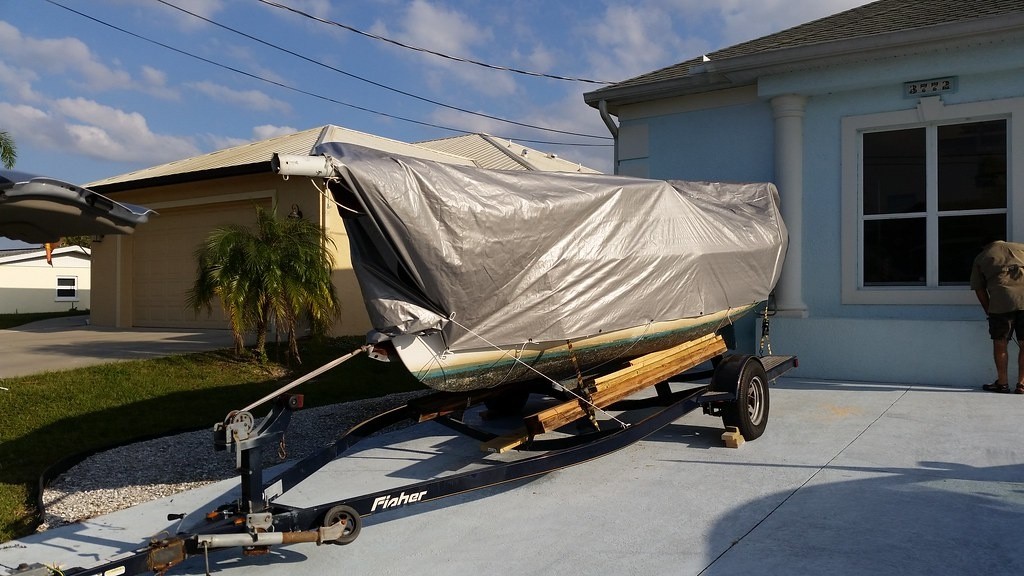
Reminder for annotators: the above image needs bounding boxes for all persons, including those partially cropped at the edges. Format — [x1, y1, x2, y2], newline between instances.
[970, 230, 1024, 394]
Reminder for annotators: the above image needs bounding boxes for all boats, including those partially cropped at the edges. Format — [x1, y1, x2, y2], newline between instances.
[267, 142, 790, 393]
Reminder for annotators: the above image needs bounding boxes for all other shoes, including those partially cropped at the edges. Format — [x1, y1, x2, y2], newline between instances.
[982, 380, 1010, 393]
[1014, 383, 1024, 393]
